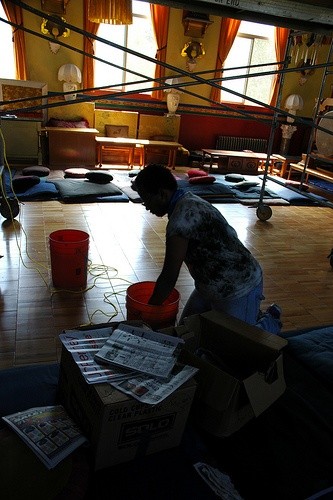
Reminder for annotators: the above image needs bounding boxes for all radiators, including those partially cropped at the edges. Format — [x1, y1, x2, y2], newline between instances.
[216, 136, 267, 152]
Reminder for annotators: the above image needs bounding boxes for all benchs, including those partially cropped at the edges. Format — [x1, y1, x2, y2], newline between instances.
[95, 136, 183, 170]
[270, 154, 301, 178]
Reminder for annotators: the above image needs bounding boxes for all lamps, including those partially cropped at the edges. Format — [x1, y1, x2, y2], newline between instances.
[284, 94, 304, 123]
[163, 78, 184, 114]
[40, 14, 70, 54]
[296, 58, 314, 87]
[181, 40, 206, 72]
[58, 64, 81, 100]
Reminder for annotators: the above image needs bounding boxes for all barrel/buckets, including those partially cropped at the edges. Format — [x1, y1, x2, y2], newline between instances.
[50, 230, 89, 291]
[127, 282, 180, 330]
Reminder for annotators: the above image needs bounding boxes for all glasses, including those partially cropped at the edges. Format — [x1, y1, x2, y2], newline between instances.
[142, 200, 153, 209]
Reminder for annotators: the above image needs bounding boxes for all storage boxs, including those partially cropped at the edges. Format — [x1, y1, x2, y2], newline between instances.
[157, 309, 288, 437]
[56, 320, 197, 471]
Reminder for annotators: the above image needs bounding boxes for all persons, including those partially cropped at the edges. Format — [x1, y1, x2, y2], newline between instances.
[130, 163, 283, 335]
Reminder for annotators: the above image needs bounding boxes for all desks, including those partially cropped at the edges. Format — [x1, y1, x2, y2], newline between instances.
[45, 127, 99, 169]
[202, 149, 278, 174]
[0, 116, 45, 166]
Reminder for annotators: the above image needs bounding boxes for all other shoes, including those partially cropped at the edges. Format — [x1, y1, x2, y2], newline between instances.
[266, 304, 283, 328]
